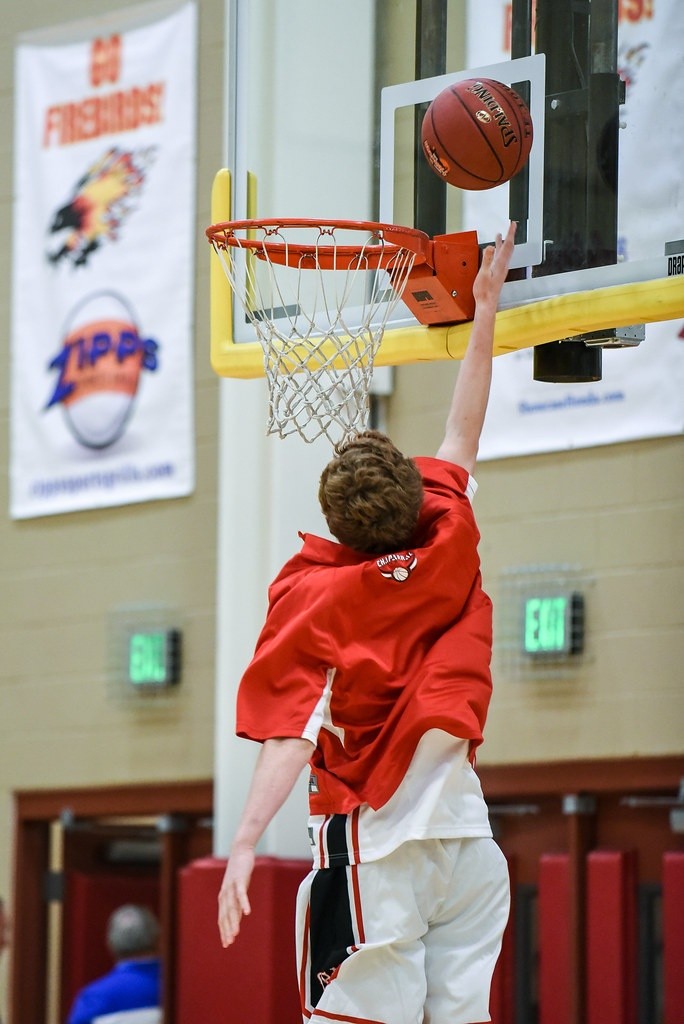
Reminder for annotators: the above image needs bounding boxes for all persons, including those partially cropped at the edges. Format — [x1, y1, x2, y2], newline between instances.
[218, 221, 518, 1024]
[68, 903, 162, 1024]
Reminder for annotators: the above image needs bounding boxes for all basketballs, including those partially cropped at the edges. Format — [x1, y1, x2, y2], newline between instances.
[421, 78, 534, 191]
